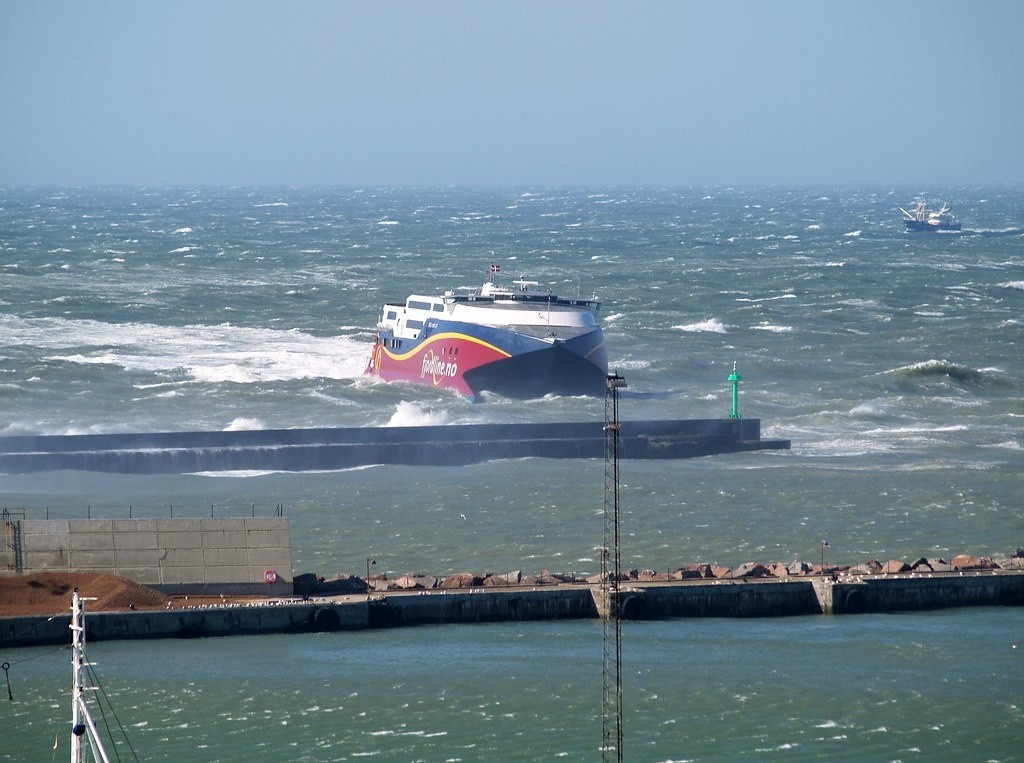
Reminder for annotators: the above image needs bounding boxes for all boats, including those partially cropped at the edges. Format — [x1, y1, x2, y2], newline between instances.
[899, 193, 962, 230]
[362, 261, 608, 405]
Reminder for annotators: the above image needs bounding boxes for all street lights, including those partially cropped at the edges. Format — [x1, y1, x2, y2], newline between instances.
[367, 557, 376, 600]
[821, 540, 829, 577]
